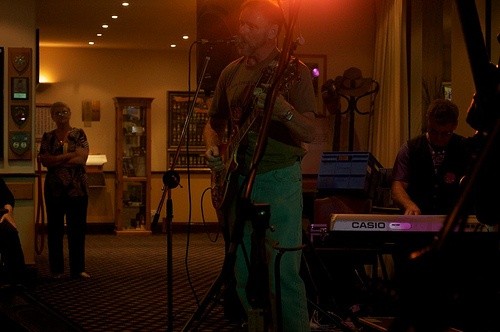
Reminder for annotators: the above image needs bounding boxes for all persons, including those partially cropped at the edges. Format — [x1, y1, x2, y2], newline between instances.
[201, 1, 320, 332]
[388, 99, 478, 320]
[0, 177, 28, 285]
[39, 101, 92, 284]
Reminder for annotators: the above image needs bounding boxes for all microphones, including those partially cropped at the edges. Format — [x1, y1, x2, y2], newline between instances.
[196, 36, 242, 46]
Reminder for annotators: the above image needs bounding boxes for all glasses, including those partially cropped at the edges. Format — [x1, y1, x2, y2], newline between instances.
[53, 111, 69, 116]
[427, 127, 453, 138]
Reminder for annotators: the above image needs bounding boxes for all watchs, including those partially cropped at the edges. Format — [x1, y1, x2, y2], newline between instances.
[278, 105, 296, 122]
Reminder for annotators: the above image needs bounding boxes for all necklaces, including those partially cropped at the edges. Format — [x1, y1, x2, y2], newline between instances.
[57, 135, 65, 144]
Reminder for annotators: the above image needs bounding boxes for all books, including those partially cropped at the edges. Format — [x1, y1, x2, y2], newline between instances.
[171, 101, 210, 168]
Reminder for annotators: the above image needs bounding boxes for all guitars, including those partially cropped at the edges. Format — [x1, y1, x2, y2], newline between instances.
[210, 55, 302, 211]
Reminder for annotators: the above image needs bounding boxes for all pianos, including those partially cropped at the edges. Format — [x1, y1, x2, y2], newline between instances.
[328, 212, 500, 233]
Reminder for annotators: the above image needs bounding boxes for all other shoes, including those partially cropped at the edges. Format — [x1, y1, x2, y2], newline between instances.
[70, 272, 90, 278]
[47, 274, 60, 279]
[4, 273, 24, 288]
